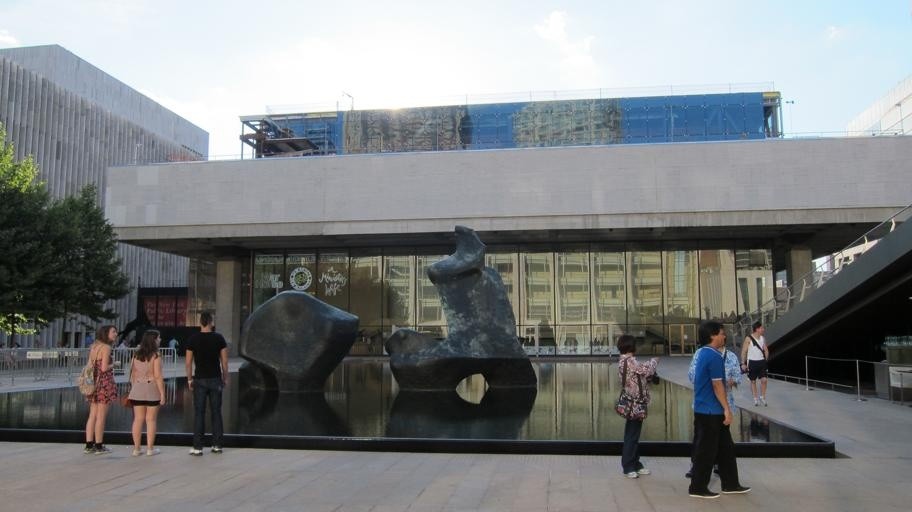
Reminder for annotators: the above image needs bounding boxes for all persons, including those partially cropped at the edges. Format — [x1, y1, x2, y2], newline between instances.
[124, 328, 166, 456]
[614, 334, 662, 478]
[686, 319, 752, 499]
[167, 337, 179, 362]
[185, 310, 228, 456]
[739, 320, 770, 407]
[79, 325, 123, 456]
[681, 318, 742, 478]
[0, 332, 140, 370]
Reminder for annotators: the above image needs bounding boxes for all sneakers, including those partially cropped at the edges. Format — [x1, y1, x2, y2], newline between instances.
[685, 469, 692, 478]
[712, 468, 720, 477]
[754, 399, 758, 406]
[211, 446, 222, 453]
[188, 447, 203, 456]
[623, 472, 639, 480]
[636, 468, 651, 475]
[688, 485, 720, 499]
[133, 449, 144, 457]
[146, 448, 161, 456]
[720, 486, 752, 495]
[760, 396, 767, 407]
[82, 446, 96, 454]
[94, 447, 112, 455]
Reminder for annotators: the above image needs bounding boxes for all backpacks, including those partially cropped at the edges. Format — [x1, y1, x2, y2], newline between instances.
[79, 341, 104, 397]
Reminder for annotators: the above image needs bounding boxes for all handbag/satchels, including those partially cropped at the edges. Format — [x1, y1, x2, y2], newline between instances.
[120, 392, 133, 408]
[615, 389, 647, 420]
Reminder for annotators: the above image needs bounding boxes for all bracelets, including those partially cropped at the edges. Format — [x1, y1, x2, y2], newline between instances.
[188, 378, 194, 383]
[740, 362, 746, 366]
[113, 362, 117, 368]
